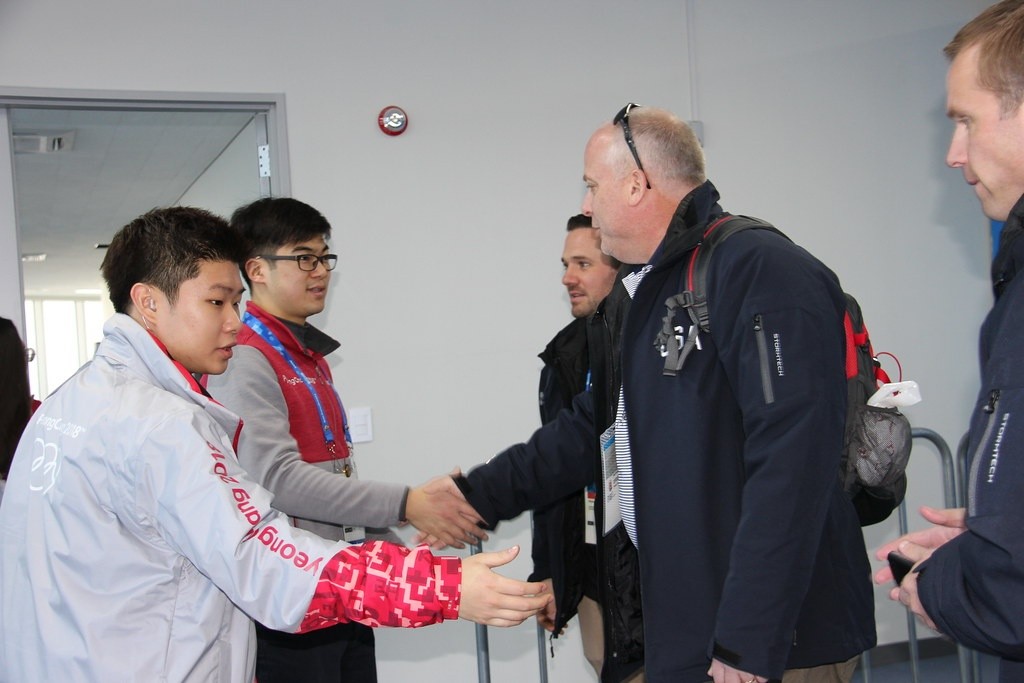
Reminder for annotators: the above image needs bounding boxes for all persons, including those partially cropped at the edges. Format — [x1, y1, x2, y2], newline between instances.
[200, 199, 489, 683]
[0, 208, 554, 683]
[873, 0, 1024, 683]
[525, 214, 647, 683]
[413, 102, 878, 683]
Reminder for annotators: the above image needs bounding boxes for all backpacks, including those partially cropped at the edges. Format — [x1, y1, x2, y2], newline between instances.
[684, 214, 912, 528]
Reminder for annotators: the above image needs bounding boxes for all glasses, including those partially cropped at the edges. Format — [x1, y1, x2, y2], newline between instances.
[614, 102, 651, 190]
[246, 253, 338, 272]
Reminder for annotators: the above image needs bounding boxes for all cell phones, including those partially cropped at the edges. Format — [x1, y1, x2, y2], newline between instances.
[888, 550, 915, 587]
